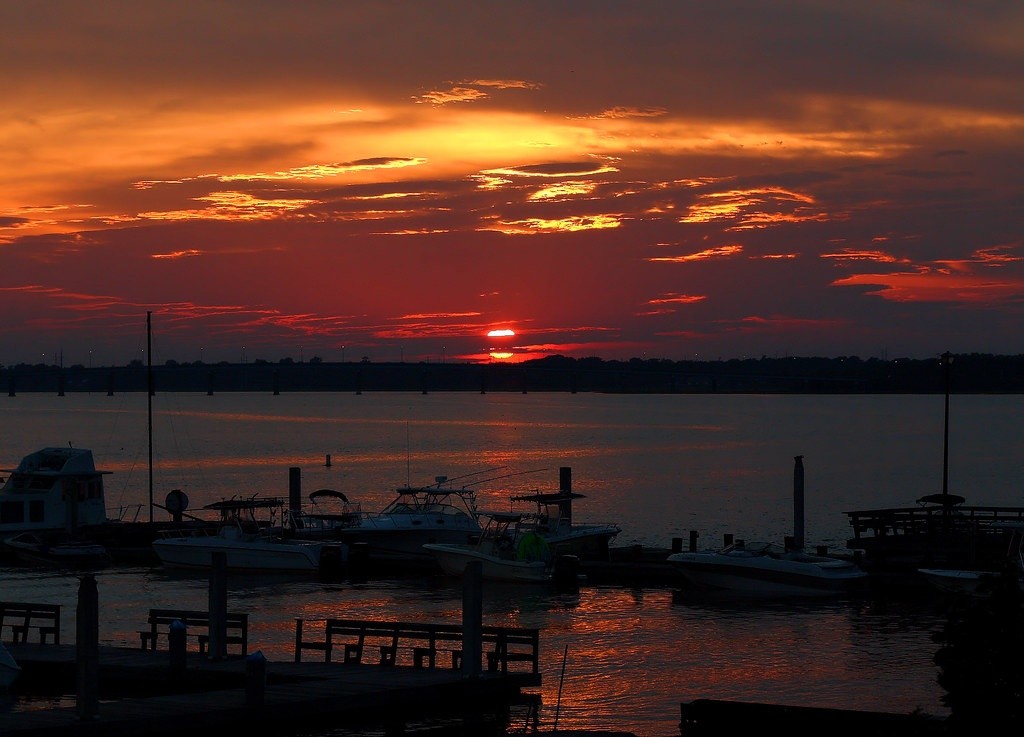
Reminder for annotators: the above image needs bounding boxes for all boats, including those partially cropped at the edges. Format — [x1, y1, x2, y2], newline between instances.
[152, 453, 856, 608]
